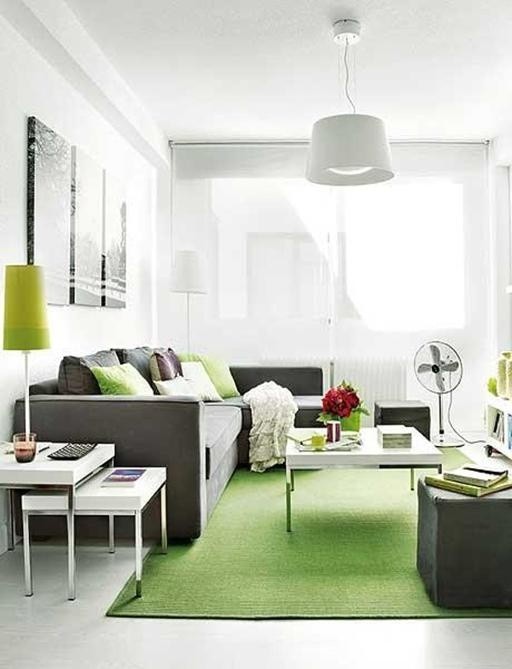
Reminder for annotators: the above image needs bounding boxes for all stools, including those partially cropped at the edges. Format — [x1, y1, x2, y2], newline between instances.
[417, 473, 512, 610]
[374, 400, 430, 467]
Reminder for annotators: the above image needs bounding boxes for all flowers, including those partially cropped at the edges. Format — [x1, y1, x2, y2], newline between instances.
[317, 380, 371, 427]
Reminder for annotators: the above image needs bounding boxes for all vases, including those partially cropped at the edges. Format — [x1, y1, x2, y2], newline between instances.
[341, 413, 361, 431]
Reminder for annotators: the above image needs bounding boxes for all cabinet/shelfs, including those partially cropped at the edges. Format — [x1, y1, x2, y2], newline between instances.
[485, 398, 512, 460]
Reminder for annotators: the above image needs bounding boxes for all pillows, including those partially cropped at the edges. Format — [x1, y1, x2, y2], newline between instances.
[150, 348, 183, 380]
[90, 362, 155, 396]
[112, 346, 155, 387]
[180, 361, 224, 403]
[58, 350, 120, 396]
[179, 352, 241, 397]
[152, 375, 202, 400]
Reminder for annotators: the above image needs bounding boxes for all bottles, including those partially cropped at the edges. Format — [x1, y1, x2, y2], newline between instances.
[495, 349, 512, 400]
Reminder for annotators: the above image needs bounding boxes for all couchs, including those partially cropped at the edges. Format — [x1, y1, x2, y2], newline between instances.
[14, 365, 324, 545]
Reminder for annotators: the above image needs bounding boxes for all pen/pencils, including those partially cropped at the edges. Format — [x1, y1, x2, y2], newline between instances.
[39, 447, 51, 452]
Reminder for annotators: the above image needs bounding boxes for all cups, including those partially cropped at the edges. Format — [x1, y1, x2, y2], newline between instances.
[13, 432, 36, 463]
[311, 434, 325, 450]
[326, 420, 342, 443]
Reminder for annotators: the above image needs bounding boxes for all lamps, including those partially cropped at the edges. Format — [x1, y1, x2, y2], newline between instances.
[171, 248, 208, 354]
[305, 18, 394, 186]
[3, 264, 52, 443]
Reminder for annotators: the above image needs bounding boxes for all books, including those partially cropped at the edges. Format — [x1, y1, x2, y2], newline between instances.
[97, 464, 150, 486]
[285, 428, 363, 452]
[492, 410, 512, 450]
[425, 472, 512, 496]
[376, 423, 411, 449]
[443, 462, 510, 488]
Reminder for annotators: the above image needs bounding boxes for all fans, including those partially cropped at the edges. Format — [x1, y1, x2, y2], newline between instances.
[413, 341, 465, 448]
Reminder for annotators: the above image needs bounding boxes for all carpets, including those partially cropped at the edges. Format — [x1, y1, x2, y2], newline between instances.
[104, 444, 512, 617]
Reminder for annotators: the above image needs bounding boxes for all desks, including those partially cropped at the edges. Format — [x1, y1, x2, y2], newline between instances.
[20, 466, 169, 600]
[0, 440, 113, 603]
